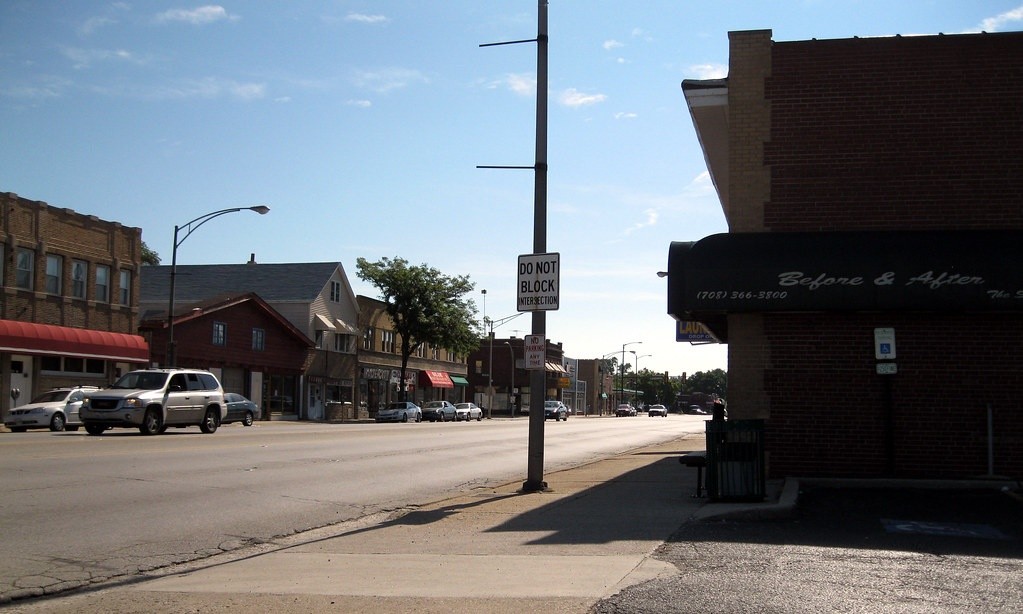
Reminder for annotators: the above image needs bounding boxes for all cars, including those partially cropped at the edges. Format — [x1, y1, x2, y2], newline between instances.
[544, 400, 568, 421]
[223, 392, 259, 427]
[615, 404, 637, 416]
[375, 402, 421, 423]
[648, 404, 668, 417]
[453, 402, 483, 421]
[422, 401, 456, 422]
[5, 386, 105, 433]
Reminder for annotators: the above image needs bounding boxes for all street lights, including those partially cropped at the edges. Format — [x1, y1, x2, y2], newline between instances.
[621, 340, 642, 405]
[599, 351, 636, 415]
[165, 205, 270, 366]
[634, 354, 652, 404]
[503, 341, 514, 418]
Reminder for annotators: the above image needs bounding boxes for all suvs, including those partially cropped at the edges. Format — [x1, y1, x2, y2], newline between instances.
[79, 367, 227, 435]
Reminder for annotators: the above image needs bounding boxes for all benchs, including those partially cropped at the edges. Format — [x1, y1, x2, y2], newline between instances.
[679, 450, 706, 498]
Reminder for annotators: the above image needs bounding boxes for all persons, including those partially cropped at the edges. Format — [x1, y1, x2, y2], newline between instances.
[419, 400, 424, 409]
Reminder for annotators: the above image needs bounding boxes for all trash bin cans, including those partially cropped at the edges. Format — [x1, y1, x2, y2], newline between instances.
[706, 420, 769, 503]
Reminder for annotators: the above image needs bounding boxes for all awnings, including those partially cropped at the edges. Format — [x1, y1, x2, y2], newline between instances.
[0, 319, 149, 362]
[335, 319, 361, 334]
[545, 363, 566, 373]
[419, 370, 453, 388]
[315, 315, 336, 330]
[450, 377, 469, 387]
